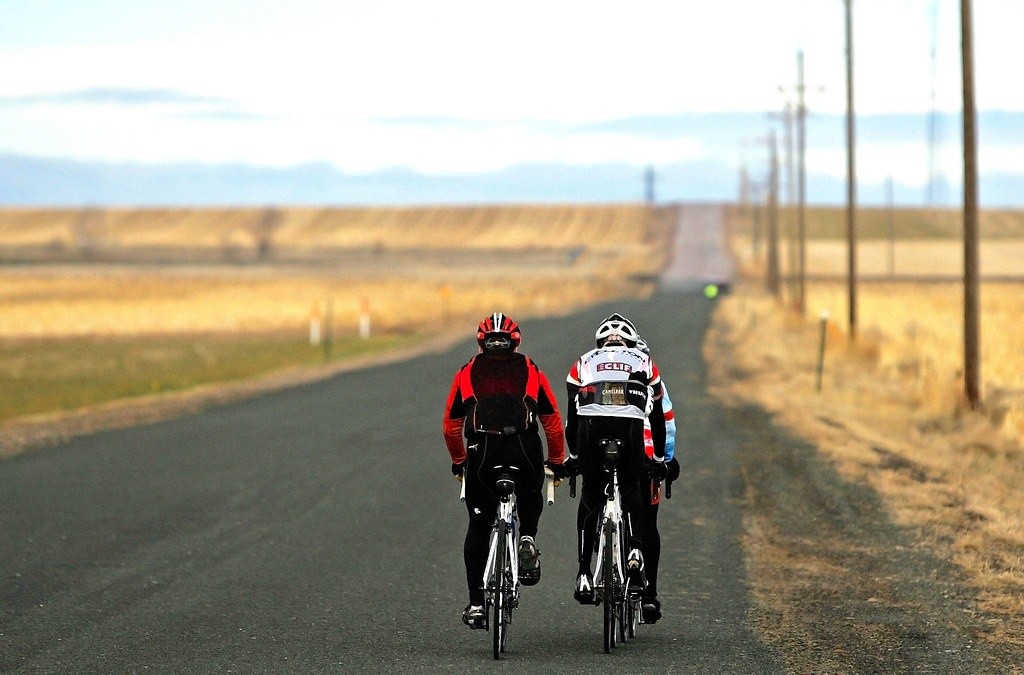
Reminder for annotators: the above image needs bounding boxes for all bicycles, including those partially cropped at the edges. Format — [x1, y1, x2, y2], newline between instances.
[565, 430, 684, 655]
[450, 453, 557, 662]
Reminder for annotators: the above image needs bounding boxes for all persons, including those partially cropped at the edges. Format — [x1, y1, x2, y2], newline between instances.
[442, 312, 564, 627]
[565, 313, 680, 623]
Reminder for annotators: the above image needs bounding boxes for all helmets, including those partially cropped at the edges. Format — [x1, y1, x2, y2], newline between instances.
[595, 313, 637, 348]
[637, 335, 650, 353]
[477, 312, 521, 352]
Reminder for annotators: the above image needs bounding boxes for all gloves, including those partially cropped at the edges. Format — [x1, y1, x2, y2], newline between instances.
[544, 460, 565, 487]
[562, 456, 582, 477]
[451, 461, 466, 482]
[646, 458, 667, 482]
[666, 456, 680, 484]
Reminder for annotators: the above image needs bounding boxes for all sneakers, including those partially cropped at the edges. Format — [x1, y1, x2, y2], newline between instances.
[574, 573, 594, 603]
[642, 601, 662, 623]
[462, 603, 486, 628]
[519, 534, 541, 586]
[627, 548, 647, 600]
